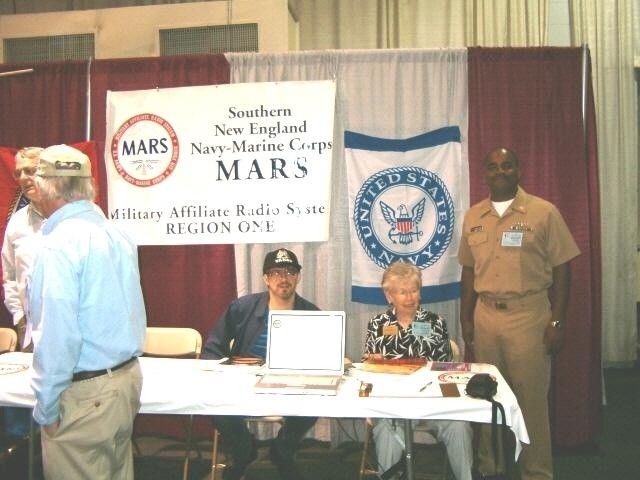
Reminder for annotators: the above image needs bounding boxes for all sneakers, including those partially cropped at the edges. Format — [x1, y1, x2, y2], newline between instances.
[222, 449, 261, 480]
[270, 441, 303, 480]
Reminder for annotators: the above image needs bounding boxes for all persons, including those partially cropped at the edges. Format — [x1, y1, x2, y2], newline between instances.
[23, 143, 149, 480]
[459, 146, 583, 479]
[1, 146, 48, 353]
[202, 248, 333, 480]
[363, 260, 478, 480]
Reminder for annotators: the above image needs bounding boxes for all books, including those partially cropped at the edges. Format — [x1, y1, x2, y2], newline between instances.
[430, 359, 473, 374]
[363, 354, 427, 375]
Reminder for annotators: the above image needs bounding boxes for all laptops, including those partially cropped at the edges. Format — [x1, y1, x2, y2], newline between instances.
[254, 310, 347, 396]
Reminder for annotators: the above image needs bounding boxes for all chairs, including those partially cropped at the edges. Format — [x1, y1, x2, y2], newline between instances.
[1, 327, 41, 479]
[132, 326, 203, 479]
[359, 341, 461, 479]
[211, 339, 285, 477]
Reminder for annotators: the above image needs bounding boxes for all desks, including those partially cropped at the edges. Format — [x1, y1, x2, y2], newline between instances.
[0, 353, 498, 476]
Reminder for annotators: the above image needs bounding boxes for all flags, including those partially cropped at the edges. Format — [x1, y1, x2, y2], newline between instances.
[2, 140, 100, 299]
[102, 80, 335, 244]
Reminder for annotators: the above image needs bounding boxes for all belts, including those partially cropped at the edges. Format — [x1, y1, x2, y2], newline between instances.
[74, 356, 137, 381]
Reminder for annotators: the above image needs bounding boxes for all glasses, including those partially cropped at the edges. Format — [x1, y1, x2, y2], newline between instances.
[265, 270, 298, 276]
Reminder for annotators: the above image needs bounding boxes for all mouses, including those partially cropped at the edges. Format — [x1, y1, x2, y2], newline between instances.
[360, 383, 373, 397]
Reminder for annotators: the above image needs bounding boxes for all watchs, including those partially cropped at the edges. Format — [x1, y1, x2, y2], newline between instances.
[550, 321, 563, 330]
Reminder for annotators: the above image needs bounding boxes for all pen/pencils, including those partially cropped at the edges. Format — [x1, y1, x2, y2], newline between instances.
[420, 382, 432, 392]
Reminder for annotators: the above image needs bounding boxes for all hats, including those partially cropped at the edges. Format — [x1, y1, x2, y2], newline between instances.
[36, 144, 91, 177]
[264, 249, 301, 273]
